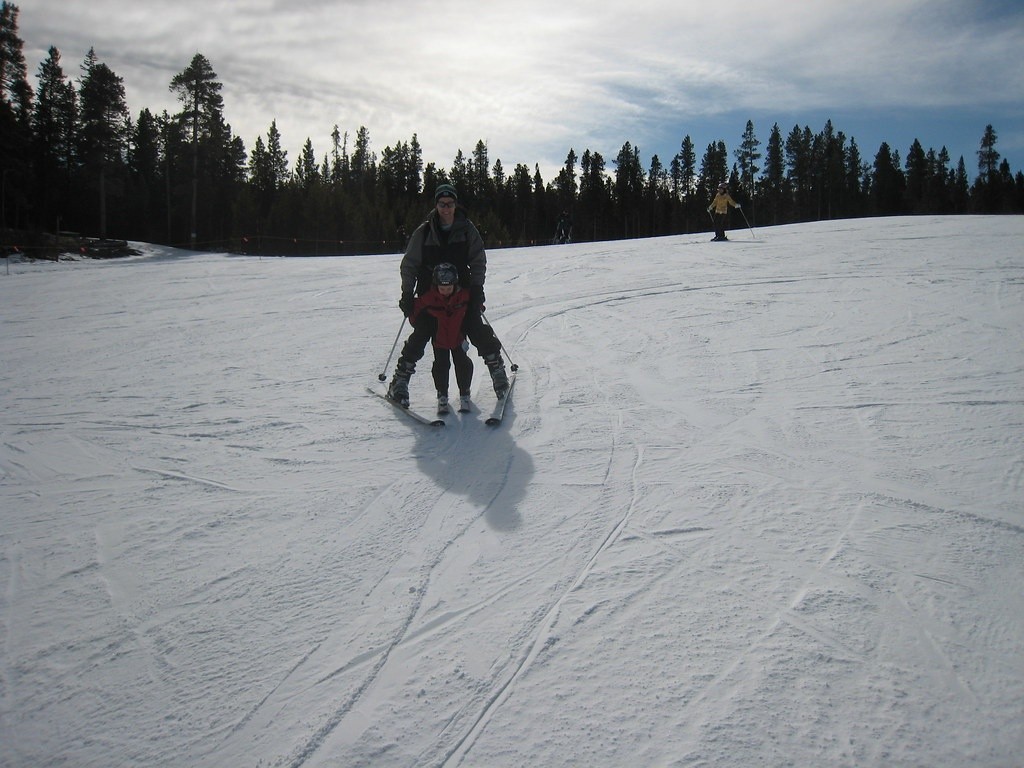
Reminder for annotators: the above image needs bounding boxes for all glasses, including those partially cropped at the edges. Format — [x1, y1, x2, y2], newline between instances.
[437, 200, 456, 208]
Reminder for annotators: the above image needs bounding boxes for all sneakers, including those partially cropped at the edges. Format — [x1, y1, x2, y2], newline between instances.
[437, 395, 449, 414]
[458, 394, 471, 413]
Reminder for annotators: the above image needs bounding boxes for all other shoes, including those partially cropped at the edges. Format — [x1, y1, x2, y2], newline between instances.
[715, 235, 725, 239]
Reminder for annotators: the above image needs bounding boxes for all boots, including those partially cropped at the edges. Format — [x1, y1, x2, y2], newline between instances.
[389, 356, 416, 408]
[485, 352, 510, 392]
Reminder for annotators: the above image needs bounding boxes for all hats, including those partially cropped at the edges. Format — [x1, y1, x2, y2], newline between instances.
[435, 184, 458, 206]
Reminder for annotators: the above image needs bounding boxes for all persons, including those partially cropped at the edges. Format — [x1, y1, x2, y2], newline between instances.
[407, 261, 487, 415]
[706, 183, 741, 241]
[389, 185, 511, 409]
[554, 209, 574, 244]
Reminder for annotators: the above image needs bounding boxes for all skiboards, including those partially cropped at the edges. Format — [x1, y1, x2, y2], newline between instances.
[366, 379, 447, 428]
[485, 375, 516, 426]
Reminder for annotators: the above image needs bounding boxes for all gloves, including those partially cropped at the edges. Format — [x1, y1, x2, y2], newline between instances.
[399, 292, 414, 317]
[735, 204, 741, 208]
[707, 206, 712, 210]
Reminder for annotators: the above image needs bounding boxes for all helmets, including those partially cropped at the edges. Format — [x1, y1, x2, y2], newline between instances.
[432, 262, 458, 287]
[718, 183, 727, 188]
